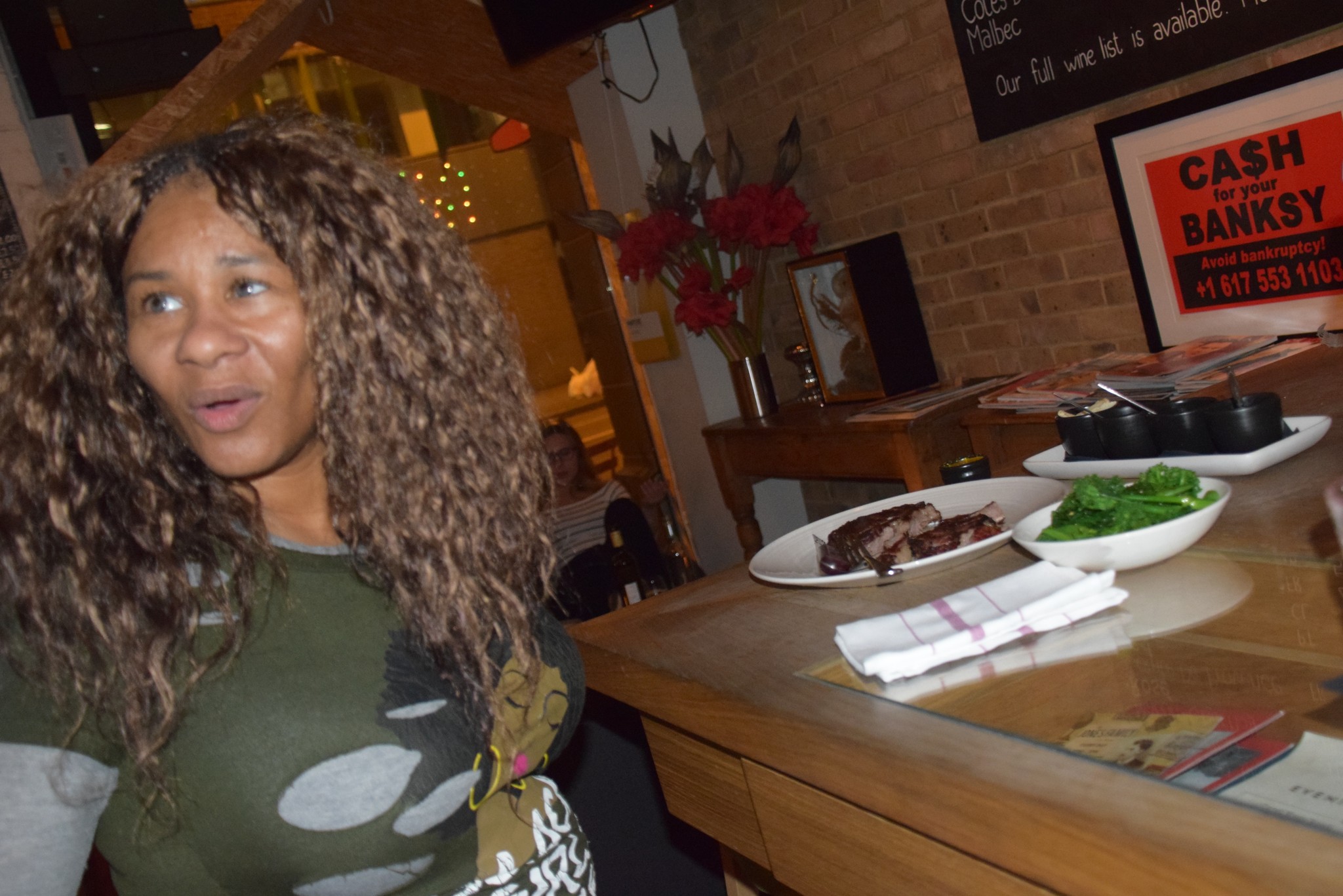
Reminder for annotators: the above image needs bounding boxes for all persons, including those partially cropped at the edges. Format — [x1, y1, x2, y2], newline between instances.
[539, 421, 666, 575]
[1, 105, 599, 896]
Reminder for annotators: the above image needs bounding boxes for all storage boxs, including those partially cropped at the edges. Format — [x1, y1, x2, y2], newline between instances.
[787, 233, 939, 404]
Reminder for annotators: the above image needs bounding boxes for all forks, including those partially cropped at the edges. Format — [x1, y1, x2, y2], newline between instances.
[842, 539, 904, 578]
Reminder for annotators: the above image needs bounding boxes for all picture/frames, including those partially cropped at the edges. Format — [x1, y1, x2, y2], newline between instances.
[1093, 44, 1343, 354]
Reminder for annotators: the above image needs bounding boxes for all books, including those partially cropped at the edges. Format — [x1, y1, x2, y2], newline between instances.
[976, 333, 1323, 414]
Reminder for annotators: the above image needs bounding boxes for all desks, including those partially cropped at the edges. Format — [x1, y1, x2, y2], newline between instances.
[963, 338, 1327, 478]
[703, 375, 1026, 558]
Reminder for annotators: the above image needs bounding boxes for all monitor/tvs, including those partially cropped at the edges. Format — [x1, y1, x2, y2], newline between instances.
[480, 0, 680, 68]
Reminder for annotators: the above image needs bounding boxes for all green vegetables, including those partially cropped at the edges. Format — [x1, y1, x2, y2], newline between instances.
[1036, 462, 1222, 540]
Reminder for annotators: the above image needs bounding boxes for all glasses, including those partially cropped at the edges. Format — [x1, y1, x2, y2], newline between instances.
[547, 448, 575, 463]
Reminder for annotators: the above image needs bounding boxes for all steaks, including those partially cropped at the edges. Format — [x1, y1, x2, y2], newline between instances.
[823, 501, 1006, 568]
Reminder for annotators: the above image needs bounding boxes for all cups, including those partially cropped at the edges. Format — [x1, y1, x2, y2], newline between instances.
[1146, 396, 1215, 456]
[1214, 391, 1283, 455]
[1094, 400, 1146, 459]
[1055, 403, 1114, 461]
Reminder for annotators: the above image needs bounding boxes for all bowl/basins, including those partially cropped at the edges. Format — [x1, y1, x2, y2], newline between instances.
[1011, 477, 1233, 571]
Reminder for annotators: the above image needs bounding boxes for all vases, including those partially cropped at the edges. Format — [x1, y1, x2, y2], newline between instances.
[728, 354, 777, 419]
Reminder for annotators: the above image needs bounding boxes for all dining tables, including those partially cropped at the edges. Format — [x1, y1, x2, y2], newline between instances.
[570, 337, 1343, 896]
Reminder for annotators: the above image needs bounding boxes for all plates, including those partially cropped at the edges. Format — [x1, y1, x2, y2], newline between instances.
[1021, 415, 1331, 476]
[748, 476, 1067, 587]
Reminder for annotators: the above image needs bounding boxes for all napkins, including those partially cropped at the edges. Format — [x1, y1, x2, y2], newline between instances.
[833, 560, 1127, 685]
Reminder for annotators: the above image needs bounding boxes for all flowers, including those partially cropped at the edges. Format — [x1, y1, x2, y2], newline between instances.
[571, 113, 819, 360]
